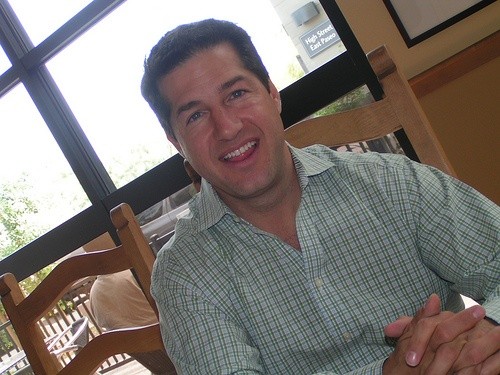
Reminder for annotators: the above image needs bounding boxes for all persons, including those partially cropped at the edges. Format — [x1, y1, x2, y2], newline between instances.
[141, 18, 500, 375]
[82, 231, 159, 331]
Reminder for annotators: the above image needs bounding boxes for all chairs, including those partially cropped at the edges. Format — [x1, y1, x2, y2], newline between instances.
[0, 42, 459, 375]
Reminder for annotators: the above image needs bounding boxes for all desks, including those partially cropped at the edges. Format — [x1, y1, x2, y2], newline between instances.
[0, 334, 57, 375]
